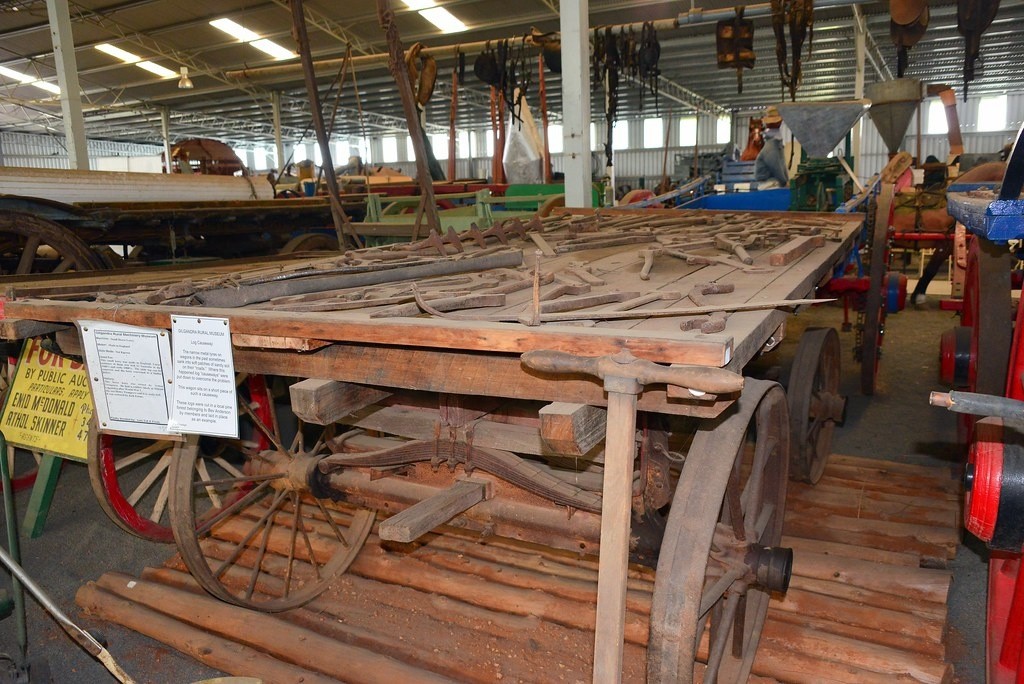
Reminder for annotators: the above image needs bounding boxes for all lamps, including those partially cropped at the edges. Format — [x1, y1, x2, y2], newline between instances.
[177, 67, 194, 88]
